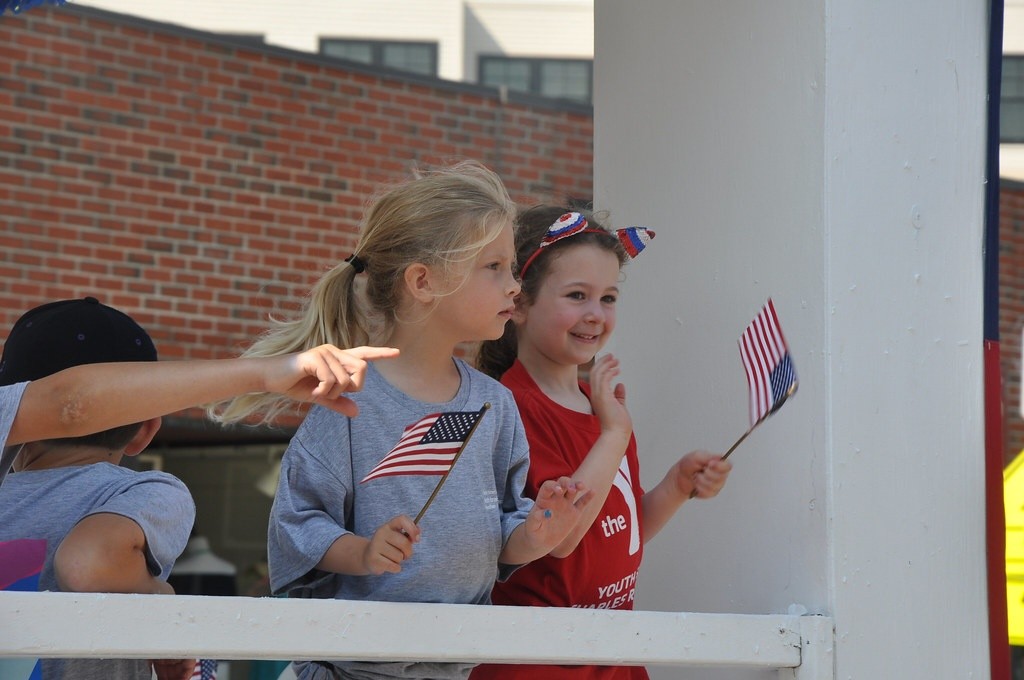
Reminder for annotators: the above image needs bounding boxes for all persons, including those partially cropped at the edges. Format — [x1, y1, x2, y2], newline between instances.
[468, 193, 735, 680]
[0, 288, 200, 680]
[189, 155, 596, 679]
[0, 334, 408, 458]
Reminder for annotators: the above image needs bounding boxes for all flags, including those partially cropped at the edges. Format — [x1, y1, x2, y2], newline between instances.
[735, 296, 798, 427]
